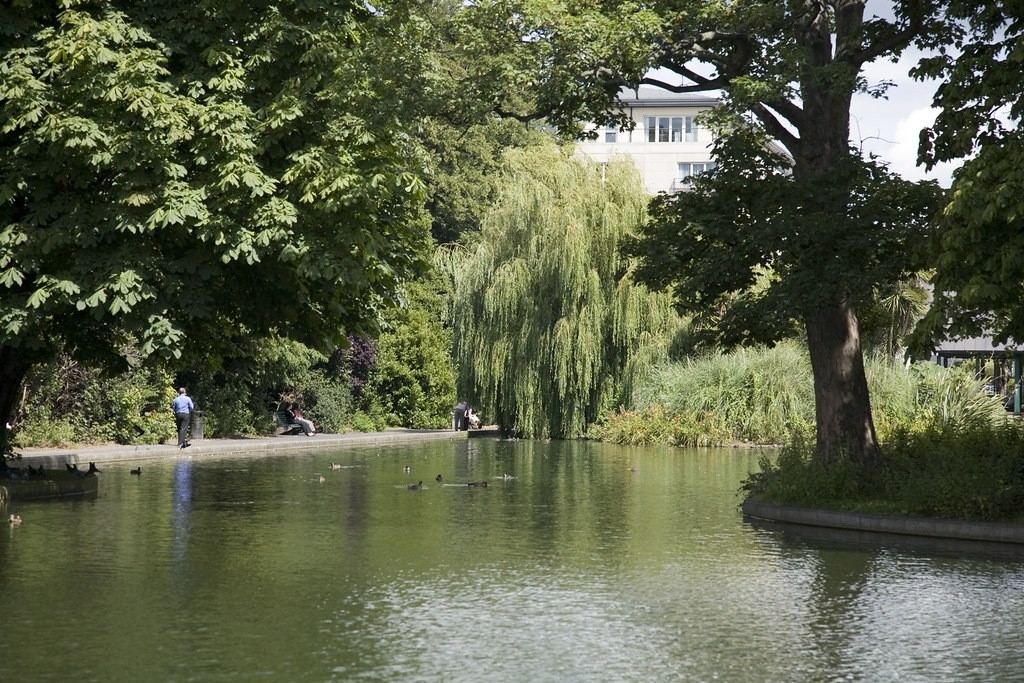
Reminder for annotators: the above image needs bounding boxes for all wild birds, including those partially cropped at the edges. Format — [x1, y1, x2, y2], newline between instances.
[468, 481, 489, 488]
[7, 514, 22, 524]
[318, 475, 324, 481]
[4, 462, 143, 481]
[435, 474, 442, 481]
[329, 462, 340, 470]
[406, 481, 423, 489]
[403, 465, 411, 472]
[631, 465, 637, 471]
[502, 473, 513, 480]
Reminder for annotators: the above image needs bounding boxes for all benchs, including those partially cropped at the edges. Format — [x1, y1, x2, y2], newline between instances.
[274, 411, 301, 435]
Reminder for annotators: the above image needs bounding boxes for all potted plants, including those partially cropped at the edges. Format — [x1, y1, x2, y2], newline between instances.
[189, 393, 204, 439]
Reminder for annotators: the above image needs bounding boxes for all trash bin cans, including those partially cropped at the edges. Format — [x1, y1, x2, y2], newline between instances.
[190, 411, 205, 437]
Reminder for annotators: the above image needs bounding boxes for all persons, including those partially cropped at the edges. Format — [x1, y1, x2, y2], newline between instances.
[172, 388, 194, 449]
[454, 401, 480, 431]
[286, 402, 316, 437]
